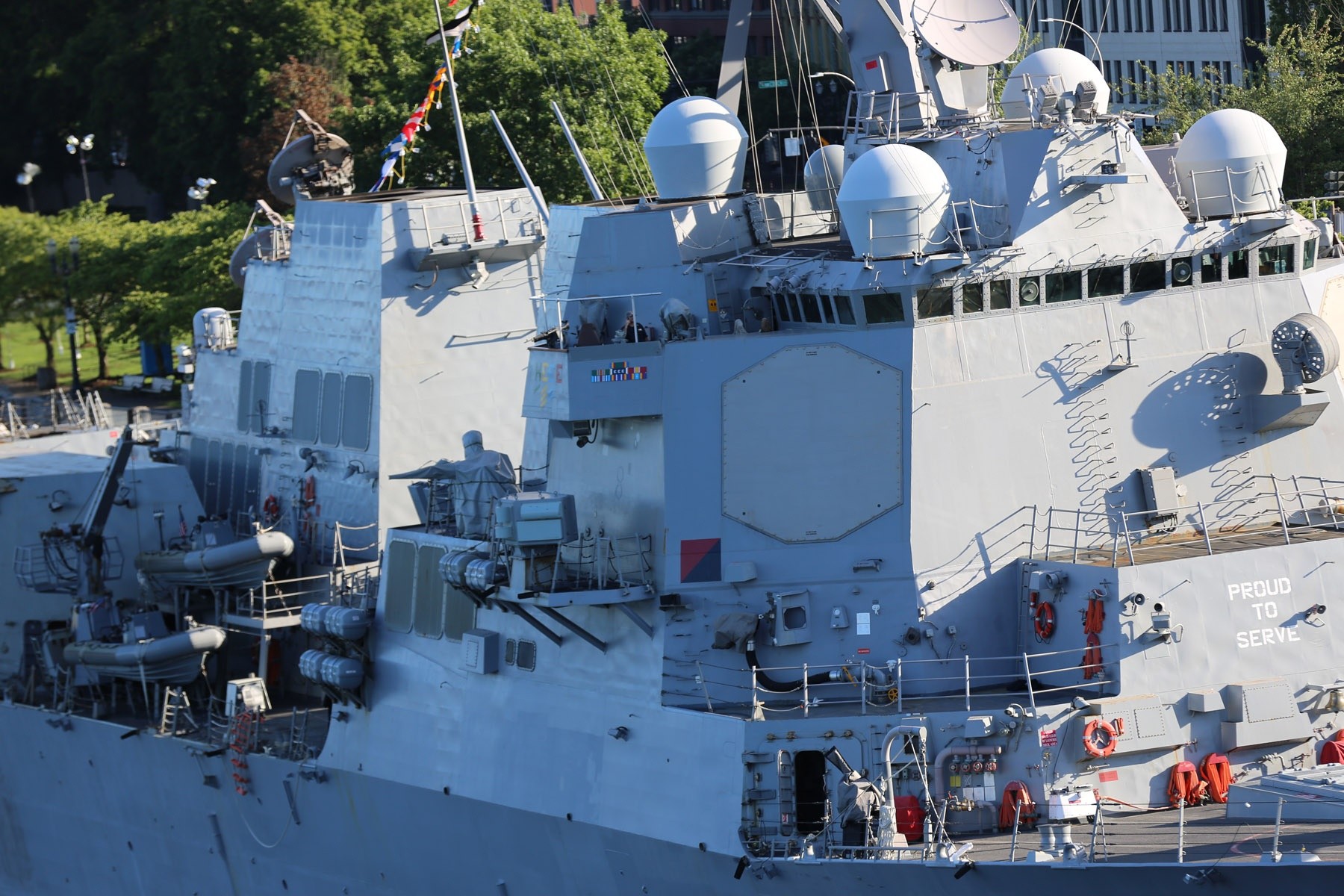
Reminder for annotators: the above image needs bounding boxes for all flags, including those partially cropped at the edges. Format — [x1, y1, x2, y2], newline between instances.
[369, 0, 482, 192]
[179, 509, 188, 537]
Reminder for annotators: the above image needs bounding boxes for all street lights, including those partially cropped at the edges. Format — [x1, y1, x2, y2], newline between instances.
[65, 133, 95, 203]
[188, 178, 215, 207]
[17, 162, 39, 213]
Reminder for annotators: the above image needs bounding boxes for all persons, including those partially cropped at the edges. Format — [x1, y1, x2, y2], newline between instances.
[5, 673, 29, 703]
[620, 311, 648, 343]
[758, 318, 772, 333]
[1214, 253, 1221, 279]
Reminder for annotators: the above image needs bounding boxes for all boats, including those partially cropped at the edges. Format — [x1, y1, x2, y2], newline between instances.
[133, 508, 296, 593]
[0, 0, 1343, 895]
[59, 601, 225, 686]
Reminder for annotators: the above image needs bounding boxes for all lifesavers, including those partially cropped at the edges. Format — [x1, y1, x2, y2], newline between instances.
[1084, 719, 1117, 757]
[1036, 601, 1053, 637]
[264, 495, 279, 524]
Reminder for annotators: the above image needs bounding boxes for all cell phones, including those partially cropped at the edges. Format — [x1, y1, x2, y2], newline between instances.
[626, 317, 629, 320]
